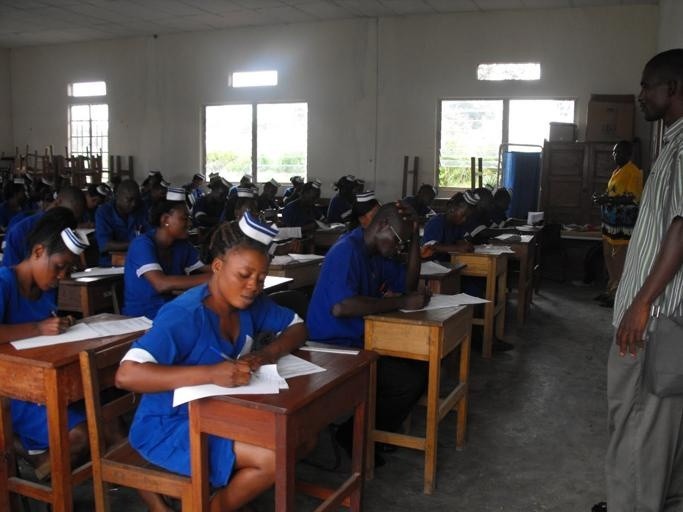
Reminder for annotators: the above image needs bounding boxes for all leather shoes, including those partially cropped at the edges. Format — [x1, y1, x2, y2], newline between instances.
[335, 423, 385, 471]
[377, 443, 399, 453]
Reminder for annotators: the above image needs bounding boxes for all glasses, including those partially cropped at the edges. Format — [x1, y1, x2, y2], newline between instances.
[388, 226, 413, 250]
[165, 214, 194, 225]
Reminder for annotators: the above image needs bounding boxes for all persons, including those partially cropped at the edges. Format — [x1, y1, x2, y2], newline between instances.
[306, 199, 433, 467]
[257, 180, 281, 210]
[1, 207, 177, 511]
[326, 175, 353, 222]
[406, 184, 437, 219]
[85, 183, 111, 209]
[592, 49, 683, 512]
[283, 179, 329, 233]
[115, 208, 308, 512]
[0, 186, 88, 267]
[122, 188, 212, 320]
[423, 189, 480, 253]
[181, 174, 205, 209]
[143, 170, 163, 191]
[0, 177, 25, 233]
[284, 176, 304, 201]
[592, 139, 643, 307]
[486, 188, 511, 230]
[95, 179, 153, 268]
[193, 178, 233, 230]
[337, 191, 382, 242]
[142, 181, 168, 222]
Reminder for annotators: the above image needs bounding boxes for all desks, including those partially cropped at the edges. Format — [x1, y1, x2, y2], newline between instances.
[401, 156, 482, 212]
[314, 228, 346, 246]
[109, 251, 128, 265]
[560, 227, 605, 265]
[473, 235, 536, 336]
[0, 313, 147, 512]
[417, 262, 467, 294]
[363, 292, 474, 495]
[267, 253, 326, 292]
[0, 146, 134, 189]
[188, 340, 379, 512]
[505, 223, 545, 294]
[171, 271, 294, 307]
[273, 238, 302, 255]
[58, 275, 111, 318]
[450, 251, 508, 359]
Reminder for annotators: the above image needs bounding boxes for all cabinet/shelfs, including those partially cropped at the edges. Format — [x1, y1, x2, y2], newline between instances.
[536, 139, 640, 235]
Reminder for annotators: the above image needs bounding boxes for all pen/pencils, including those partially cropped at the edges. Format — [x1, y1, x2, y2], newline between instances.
[49, 310, 58, 316]
[210, 346, 258, 379]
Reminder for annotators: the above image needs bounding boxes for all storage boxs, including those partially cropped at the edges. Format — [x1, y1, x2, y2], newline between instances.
[549, 122, 577, 144]
[584, 92, 635, 142]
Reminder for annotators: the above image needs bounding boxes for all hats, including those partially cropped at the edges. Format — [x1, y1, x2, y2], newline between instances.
[356, 189, 377, 203]
[165, 184, 187, 202]
[239, 209, 282, 248]
[195, 171, 206, 181]
[148, 169, 157, 178]
[355, 179, 366, 185]
[345, 174, 356, 183]
[96, 184, 110, 199]
[270, 179, 281, 189]
[61, 226, 91, 256]
[235, 187, 255, 198]
[218, 179, 233, 188]
[312, 177, 322, 190]
[462, 187, 480, 206]
[160, 180, 171, 188]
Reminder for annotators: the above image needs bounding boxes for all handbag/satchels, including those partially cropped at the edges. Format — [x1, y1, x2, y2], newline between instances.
[638, 143, 683, 399]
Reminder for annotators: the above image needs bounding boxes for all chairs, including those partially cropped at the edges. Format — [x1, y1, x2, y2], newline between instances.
[79, 331, 192, 512]
[267, 290, 307, 324]
[111, 274, 125, 315]
